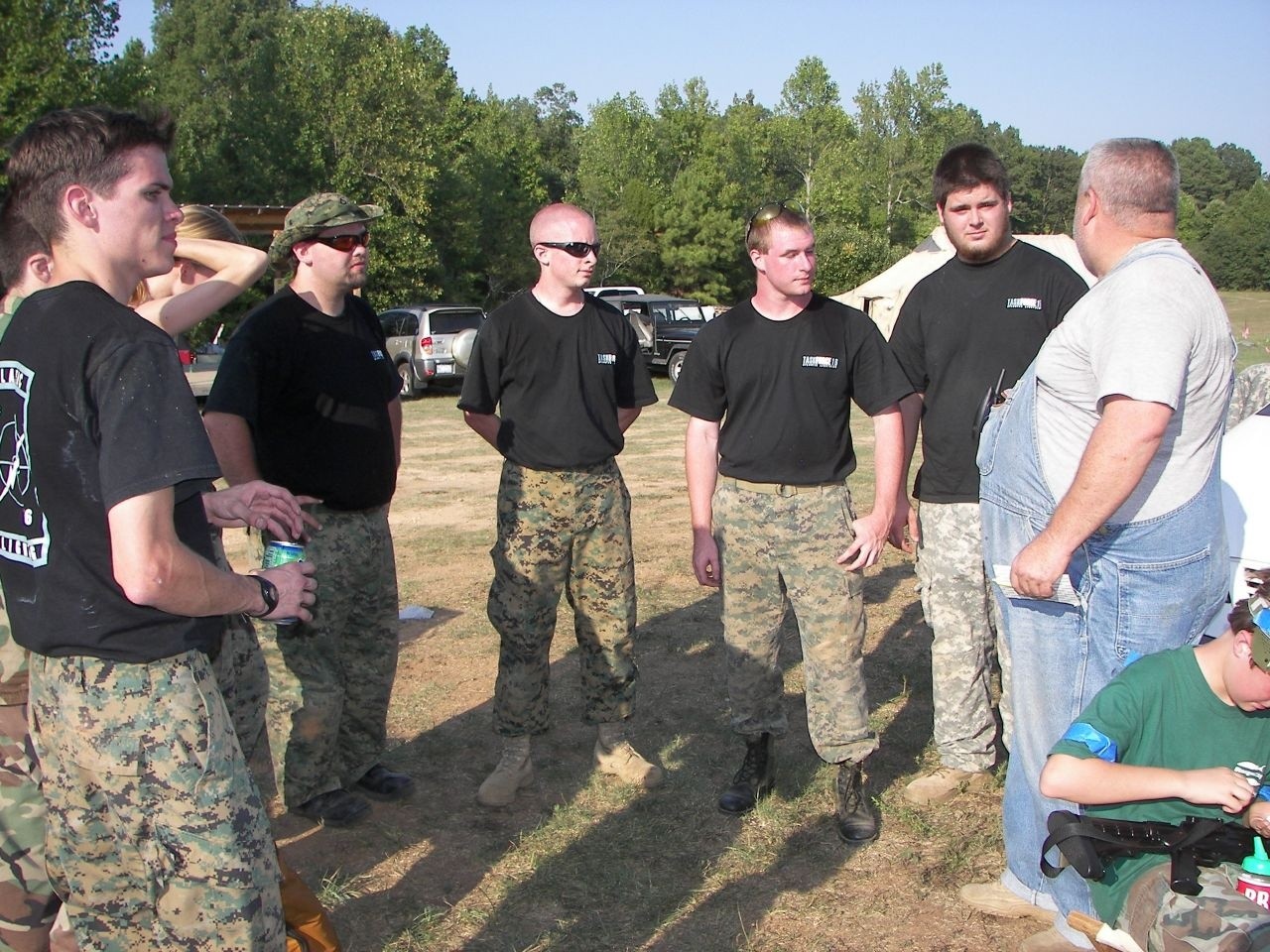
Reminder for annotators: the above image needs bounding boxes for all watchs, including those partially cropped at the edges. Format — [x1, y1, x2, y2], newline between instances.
[245, 576, 278, 619]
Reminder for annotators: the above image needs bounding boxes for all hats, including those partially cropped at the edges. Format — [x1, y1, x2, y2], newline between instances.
[268, 192, 385, 271]
[1246, 593, 1270, 671]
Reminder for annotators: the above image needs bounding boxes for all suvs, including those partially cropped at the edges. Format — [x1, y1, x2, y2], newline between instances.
[598, 292, 723, 385]
[377, 303, 489, 401]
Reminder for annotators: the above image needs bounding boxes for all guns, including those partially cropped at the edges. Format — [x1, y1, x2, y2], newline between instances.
[1045, 808, 1270, 897]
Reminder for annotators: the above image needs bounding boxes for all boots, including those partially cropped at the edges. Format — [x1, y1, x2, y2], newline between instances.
[714, 733, 770, 814]
[592, 721, 664, 786]
[479, 732, 533, 807]
[835, 763, 876, 843]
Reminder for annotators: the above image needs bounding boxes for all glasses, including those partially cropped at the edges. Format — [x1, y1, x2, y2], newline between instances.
[535, 242, 601, 260]
[744, 200, 804, 246]
[305, 230, 371, 250]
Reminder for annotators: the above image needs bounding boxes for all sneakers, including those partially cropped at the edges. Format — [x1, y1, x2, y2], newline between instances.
[961, 881, 1059, 924]
[903, 765, 991, 804]
[354, 767, 415, 798]
[1019, 926, 1094, 952]
[290, 789, 372, 827]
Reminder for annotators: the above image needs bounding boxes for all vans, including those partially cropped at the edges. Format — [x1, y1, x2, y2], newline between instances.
[582, 286, 646, 317]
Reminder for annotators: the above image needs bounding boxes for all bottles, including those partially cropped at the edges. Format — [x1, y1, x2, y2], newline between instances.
[1236, 836, 1270, 913]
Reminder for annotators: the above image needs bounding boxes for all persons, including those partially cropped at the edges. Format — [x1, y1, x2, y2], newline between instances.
[959, 137, 1238, 952]
[668, 197, 915, 845]
[0, 101, 319, 952]
[1040, 580, 1270, 952]
[201, 194, 416, 823]
[456, 203, 667, 807]
[887, 145, 1090, 804]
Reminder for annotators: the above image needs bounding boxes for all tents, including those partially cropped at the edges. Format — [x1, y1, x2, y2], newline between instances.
[827, 226, 1099, 343]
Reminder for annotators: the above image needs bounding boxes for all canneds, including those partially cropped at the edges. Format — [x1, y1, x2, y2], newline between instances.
[262, 540, 304, 625]
